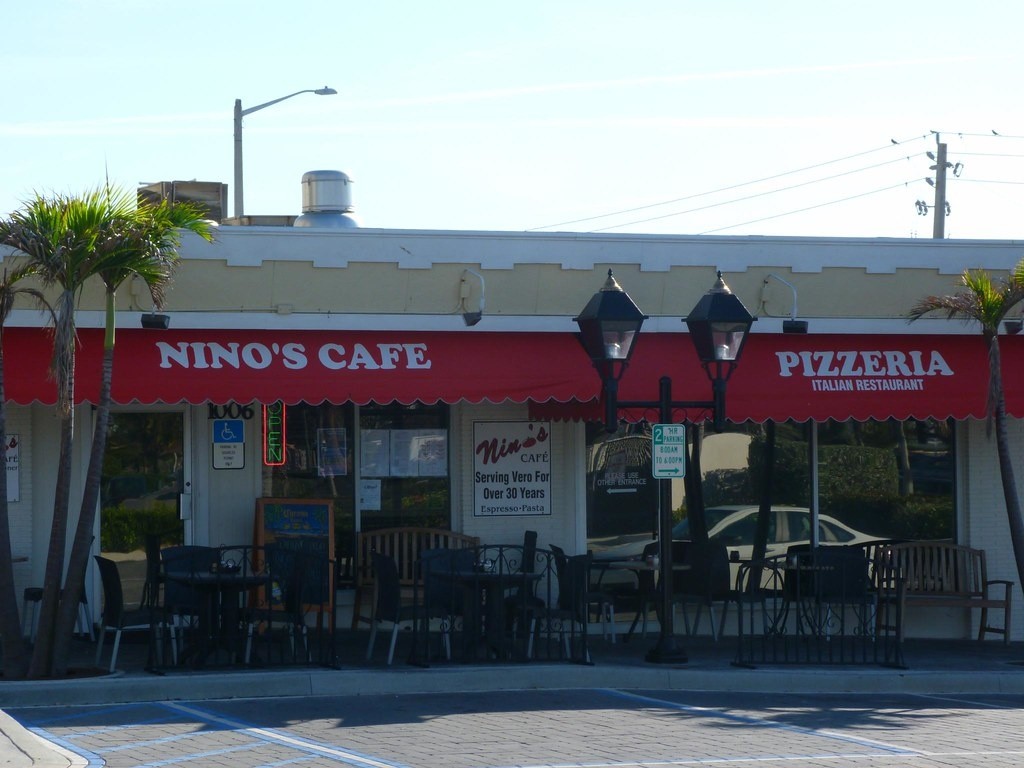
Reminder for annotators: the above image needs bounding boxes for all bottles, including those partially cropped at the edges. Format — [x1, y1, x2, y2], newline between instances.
[265, 563, 282, 604]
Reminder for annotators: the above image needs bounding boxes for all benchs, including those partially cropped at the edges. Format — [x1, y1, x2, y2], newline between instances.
[871, 541, 1012, 671]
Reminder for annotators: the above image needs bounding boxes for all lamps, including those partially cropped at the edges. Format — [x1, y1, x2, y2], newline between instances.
[130, 274, 171, 330]
[461, 267, 485, 324]
[765, 273, 809, 334]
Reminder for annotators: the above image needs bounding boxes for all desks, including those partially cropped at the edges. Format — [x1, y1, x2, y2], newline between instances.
[161, 567, 276, 665]
[745, 561, 837, 665]
[588, 560, 689, 648]
[434, 563, 545, 666]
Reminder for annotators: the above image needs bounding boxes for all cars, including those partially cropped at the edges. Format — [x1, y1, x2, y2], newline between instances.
[588, 505, 904, 594]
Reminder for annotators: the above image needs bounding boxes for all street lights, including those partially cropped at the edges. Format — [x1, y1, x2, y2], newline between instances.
[232, 87, 337, 209]
[577, 267, 759, 662]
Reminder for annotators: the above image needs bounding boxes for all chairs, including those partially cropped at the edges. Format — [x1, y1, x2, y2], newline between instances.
[24, 536, 909, 670]
